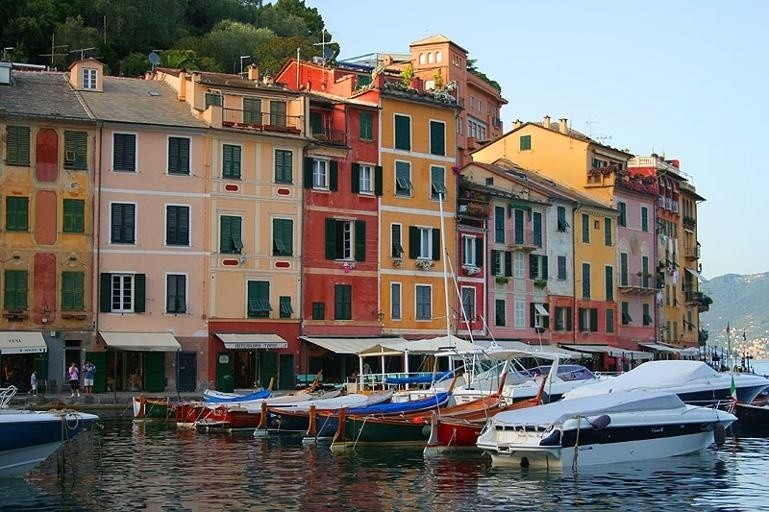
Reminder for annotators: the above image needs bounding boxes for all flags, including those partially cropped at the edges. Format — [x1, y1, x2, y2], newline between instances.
[727, 322, 731, 333]
[743, 331, 747, 341]
[729, 375, 738, 412]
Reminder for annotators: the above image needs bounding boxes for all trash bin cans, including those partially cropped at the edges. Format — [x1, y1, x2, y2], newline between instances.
[223, 375, 233, 393]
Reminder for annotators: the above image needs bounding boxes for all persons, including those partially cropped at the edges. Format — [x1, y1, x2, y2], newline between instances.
[27, 369, 39, 397]
[69, 361, 81, 398]
[349, 372, 357, 381]
[82, 360, 96, 395]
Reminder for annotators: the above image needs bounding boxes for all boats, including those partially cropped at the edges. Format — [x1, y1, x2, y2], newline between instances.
[0, 382, 101, 478]
[131, 189, 768, 458]
[475, 385, 739, 471]
